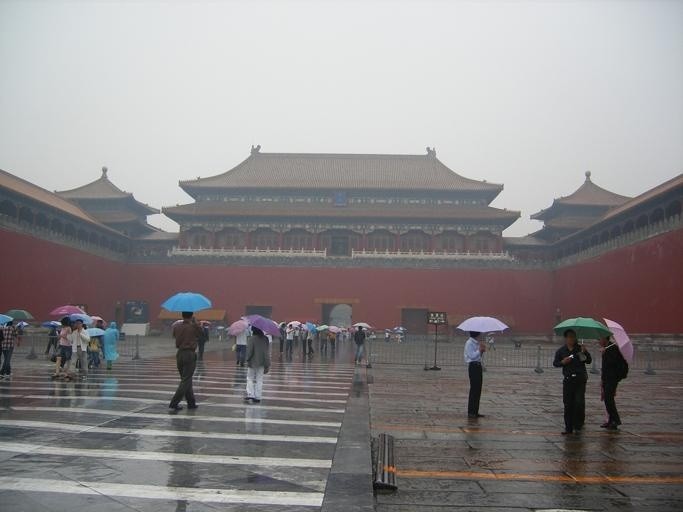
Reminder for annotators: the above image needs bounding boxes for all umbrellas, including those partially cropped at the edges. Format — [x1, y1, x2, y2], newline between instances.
[602, 316, 633, 369]
[487, 332, 496, 335]
[158, 291, 212, 313]
[551, 316, 614, 354]
[455, 316, 510, 332]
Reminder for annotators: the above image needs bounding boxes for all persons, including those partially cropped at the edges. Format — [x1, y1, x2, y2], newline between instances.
[552, 329, 592, 436]
[0, 303, 121, 382]
[463, 330, 486, 422]
[487, 335, 495, 351]
[1, 383, 13, 415]
[243, 326, 270, 404]
[597, 335, 628, 430]
[243, 404, 265, 485]
[225, 313, 409, 363]
[166, 311, 201, 413]
[599, 436, 633, 511]
[163, 416, 200, 511]
[48, 383, 105, 415]
[557, 440, 589, 511]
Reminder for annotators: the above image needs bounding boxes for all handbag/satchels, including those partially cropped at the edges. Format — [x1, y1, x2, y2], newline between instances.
[91, 343, 99, 352]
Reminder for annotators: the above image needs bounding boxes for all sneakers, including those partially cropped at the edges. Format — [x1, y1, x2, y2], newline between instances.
[468, 412, 484, 419]
[52, 373, 88, 382]
[187, 404, 199, 409]
[169, 404, 183, 410]
[600, 421, 618, 429]
[561, 428, 582, 435]
[0, 371, 14, 378]
[244, 396, 260, 403]
